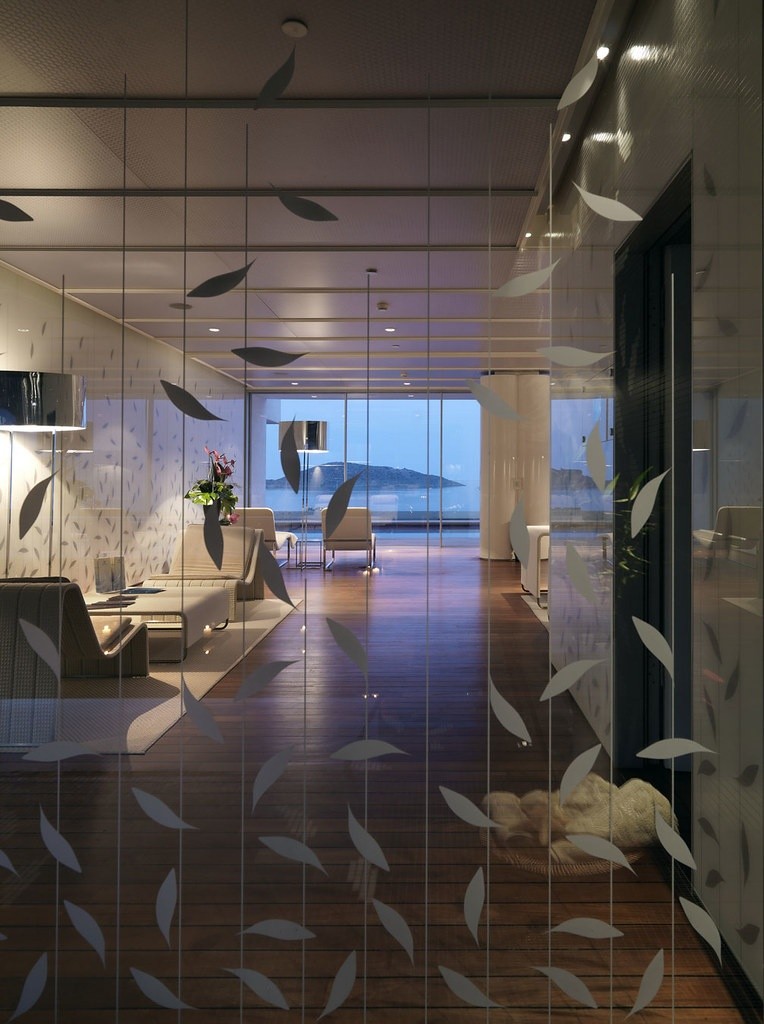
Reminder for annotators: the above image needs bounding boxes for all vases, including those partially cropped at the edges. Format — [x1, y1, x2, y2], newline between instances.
[204, 499, 220, 523]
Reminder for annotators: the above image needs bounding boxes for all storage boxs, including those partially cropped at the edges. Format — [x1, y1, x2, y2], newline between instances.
[94, 556, 125, 593]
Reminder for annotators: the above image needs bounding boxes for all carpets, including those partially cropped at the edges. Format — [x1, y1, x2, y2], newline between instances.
[521, 595, 549, 634]
[0, 599, 304, 755]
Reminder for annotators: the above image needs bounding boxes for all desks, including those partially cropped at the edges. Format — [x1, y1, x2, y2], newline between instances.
[550, 508, 614, 562]
[83, 587, 228, 664]
[296, 539, 322, 567]
[521, 525, 550, 608]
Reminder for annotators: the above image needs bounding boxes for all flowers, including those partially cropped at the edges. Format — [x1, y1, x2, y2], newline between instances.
[183, 446, 238, 526]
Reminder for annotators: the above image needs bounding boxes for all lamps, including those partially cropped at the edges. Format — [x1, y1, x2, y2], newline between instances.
[279, 421, 327, 571]
[0, 370, 85, 576]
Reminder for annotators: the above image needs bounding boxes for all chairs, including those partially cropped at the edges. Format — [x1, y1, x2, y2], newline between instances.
[322, 507, 376, 570]
[231, 508, 298, 568]
[694, 507, 764, 582]
[140, 523, 263, 630]
[0, 576, 149, 748]
